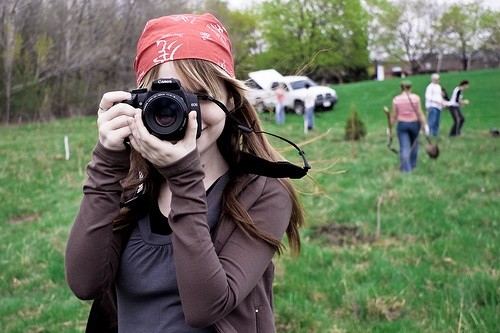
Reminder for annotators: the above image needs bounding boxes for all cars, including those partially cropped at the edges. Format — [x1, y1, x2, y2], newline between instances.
[242, 68, 338, 117]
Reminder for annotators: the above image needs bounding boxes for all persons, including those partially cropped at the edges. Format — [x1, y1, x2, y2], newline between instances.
[448, 81, 471, 136]
[275, 84, 287, 125]
[303, 84, 317, 132]
[387, 80, 430, 175]
[66, 13, 307, 333]
[425, 73, 451, 137]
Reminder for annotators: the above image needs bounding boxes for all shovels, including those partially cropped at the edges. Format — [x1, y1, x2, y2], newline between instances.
[385, 106, 398, 154]
[402, 82, 439, 158]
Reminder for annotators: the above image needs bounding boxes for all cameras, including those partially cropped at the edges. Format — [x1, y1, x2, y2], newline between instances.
[121, 77, 201, 142]
